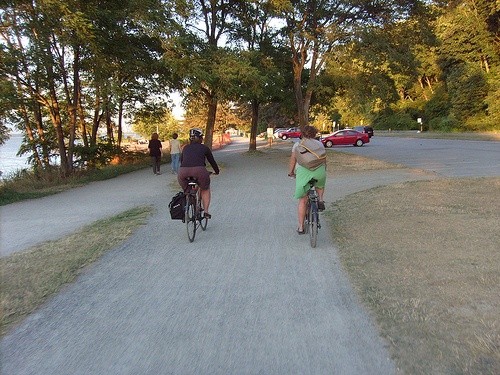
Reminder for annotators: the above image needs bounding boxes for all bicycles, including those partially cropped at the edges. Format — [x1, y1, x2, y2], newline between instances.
[185, 171, 217, 243]
[288, 173, 322, 248]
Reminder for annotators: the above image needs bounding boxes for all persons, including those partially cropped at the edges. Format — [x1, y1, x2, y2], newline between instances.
[148, 133, 162, 175]
[171, 133, 182, 175]
[178, 129, 219, 218]
[289, 126, 327, 235]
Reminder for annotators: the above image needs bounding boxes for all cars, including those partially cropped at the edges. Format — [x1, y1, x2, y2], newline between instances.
[274, 127, 302, 140]
[321, 126, 373, 148]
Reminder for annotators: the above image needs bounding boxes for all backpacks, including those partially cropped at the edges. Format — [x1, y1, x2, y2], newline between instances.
[168, 192, 186, 219]
[294, 138, 327, 168]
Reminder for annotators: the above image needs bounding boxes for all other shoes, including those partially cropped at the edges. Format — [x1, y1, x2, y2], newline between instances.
[203, 212, 211, 218]
[297, 225, 304, 234]
[318, 201, 325, 210]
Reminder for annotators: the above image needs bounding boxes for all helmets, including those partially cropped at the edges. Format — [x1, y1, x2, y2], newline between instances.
[189, 129, 204, 137]
